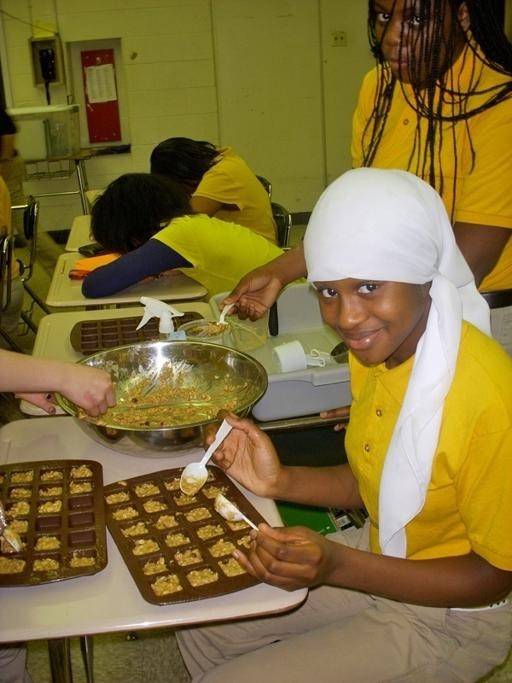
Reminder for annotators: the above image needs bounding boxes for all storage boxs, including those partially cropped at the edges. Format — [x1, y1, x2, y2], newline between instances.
[210, 284, 352, 422]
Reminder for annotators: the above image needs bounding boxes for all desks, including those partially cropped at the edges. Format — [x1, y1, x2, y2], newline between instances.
[18, 153, 99, 221]
[0, 416, 312, 682]
[22, 298, 223, 420]
[63, 213, 97, 254]
[44, 251, 208, 313]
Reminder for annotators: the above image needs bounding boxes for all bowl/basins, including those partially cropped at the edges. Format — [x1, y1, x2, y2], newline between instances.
[272, 340, 306, 372]
[55, 340, 268, 459]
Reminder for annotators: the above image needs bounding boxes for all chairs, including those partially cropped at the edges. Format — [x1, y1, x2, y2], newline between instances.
[2, 196, 49, 417]
[254, 173, 299, 338]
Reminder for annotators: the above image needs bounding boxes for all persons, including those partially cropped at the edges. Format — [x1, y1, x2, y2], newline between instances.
[220, 0, 512, 352]
[0, 64, 66, 353]
[172, 167, 512, 683]
[82, 138, 306, 301]
[0, 347, 116, 682]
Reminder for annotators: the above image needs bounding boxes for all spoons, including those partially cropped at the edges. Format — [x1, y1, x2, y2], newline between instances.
[180, 418, 234, 496]
[210, 300, 234, 325]
[215, 496, 256, 528]
